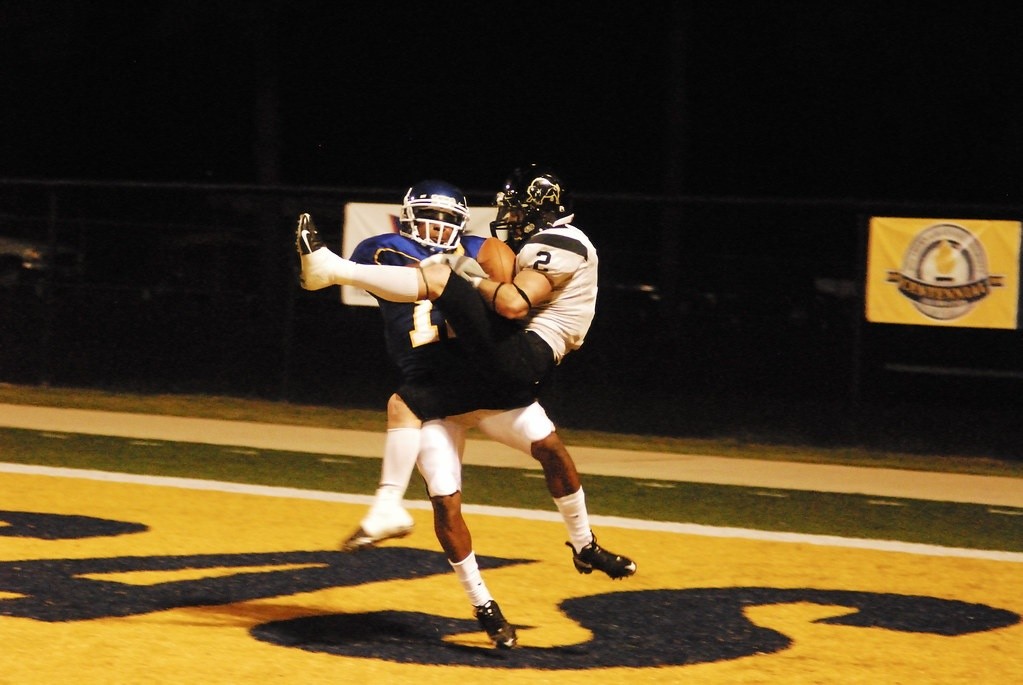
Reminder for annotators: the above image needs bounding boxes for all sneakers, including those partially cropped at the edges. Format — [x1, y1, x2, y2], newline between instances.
[295, 212, 337, 291]
[564, 529, 637, 579]
[472, 600, 515, 648]
[345, 514, 411, 552]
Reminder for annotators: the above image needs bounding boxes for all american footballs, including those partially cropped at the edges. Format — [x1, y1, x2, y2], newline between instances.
[476, 236, 518, 286]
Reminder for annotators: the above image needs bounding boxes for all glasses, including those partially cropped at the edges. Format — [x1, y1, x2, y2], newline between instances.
[416, 208, 459, 224]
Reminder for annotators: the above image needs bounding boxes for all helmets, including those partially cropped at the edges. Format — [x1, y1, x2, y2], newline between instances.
[399, 179, 469, 257]
[490, 157, 573, 255]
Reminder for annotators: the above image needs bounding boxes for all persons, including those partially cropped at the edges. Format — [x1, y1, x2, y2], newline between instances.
[296, 164, 599, 552]
[349, 181, 637, 649]
